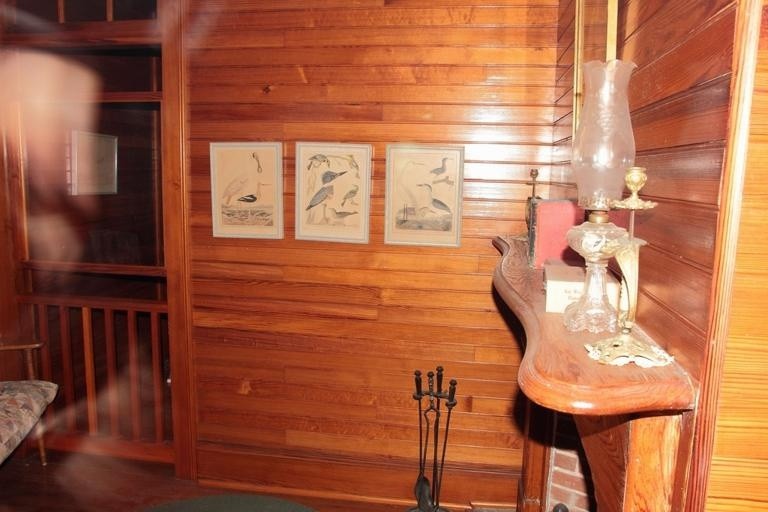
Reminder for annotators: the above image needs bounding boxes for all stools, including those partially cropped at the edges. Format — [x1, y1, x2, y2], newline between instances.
[0, 340, 59, 466]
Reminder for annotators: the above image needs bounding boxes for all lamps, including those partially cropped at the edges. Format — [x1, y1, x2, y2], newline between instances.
[564, 59, 638, 334]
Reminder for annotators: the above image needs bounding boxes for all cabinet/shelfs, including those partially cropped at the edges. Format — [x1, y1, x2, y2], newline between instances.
[490, 235, 693, 512]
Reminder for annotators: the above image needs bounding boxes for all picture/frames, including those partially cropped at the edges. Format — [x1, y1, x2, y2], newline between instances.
[209, 142, 284, 240]
[384, 143, 465, 247]
[294, 141, 372, 244]
[72, 130, 118, 196]
